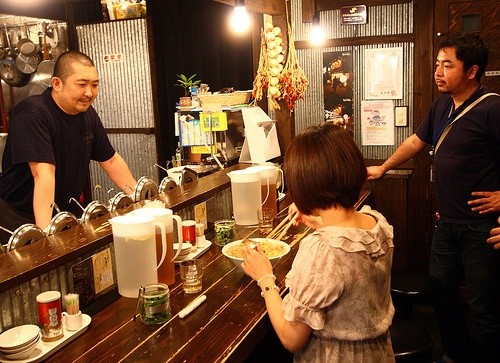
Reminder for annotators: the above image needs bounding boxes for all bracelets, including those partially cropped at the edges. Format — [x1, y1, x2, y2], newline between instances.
[261, 285, 280, 297]
[257, 274, 276, 286]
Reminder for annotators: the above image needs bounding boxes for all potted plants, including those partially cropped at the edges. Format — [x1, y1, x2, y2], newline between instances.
[175, 74, 202, 107]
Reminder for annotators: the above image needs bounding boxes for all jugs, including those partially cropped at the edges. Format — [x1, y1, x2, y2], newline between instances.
[246, 165, 284, 218]
[226, 170, 270, 225]
[108, 215, 167, 299]
[124, 207, 183, 285]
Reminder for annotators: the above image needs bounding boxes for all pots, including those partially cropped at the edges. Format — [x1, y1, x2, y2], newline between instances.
[0, 23, 38, 87]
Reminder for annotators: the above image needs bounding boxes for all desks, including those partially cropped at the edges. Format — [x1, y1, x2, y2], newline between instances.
[50, 177, 378, 363]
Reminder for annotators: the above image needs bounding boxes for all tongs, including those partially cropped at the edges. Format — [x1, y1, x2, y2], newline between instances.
[134, 286, 146, 321]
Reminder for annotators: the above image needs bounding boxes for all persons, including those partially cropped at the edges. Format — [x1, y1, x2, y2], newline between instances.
[0, 51, 138, 253]
[366, 31, 500, 363]
[241, 122, 395, 363]
[487, 217, 500, 249]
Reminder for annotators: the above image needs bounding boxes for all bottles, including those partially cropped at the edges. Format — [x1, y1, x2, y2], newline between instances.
[215, 218, 236, 245]
[139, 283, 172, 325]
[176, 141, 181, 166]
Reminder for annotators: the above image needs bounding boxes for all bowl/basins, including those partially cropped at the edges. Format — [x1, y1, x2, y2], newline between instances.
[173, 243, 192, 261]
[0, 325, 41, 359]
[222, 238, 291, 267]
[167, 165, 213, 186]
[112, 0, 147, 20]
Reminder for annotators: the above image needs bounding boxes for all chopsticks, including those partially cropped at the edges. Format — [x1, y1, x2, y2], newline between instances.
[265, 209, 299, 241]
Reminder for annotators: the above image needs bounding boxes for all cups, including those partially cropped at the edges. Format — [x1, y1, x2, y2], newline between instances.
[257, 208, 274, 232]
[62, 310, 82, 331]
[196, 235, 206, 248]
[179, 259, 203, 294]
